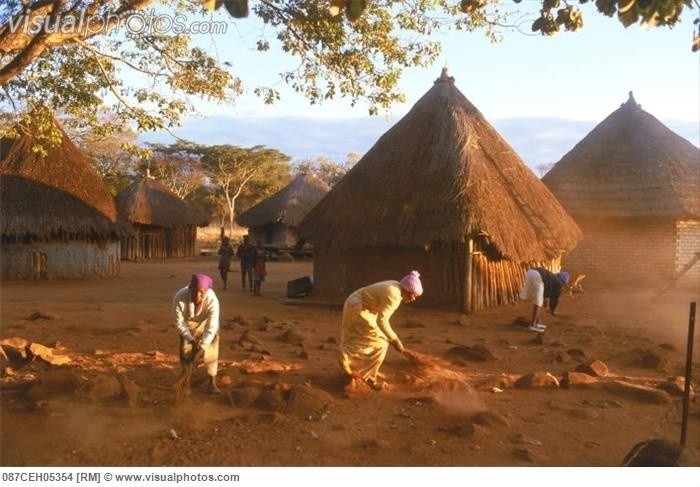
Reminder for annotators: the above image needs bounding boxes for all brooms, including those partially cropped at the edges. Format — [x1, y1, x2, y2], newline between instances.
[171, 339, 202, 397]
[545, 308, 576, 324]
[385, 339, 447, 380]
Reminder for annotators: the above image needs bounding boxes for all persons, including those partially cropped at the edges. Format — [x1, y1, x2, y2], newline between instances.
[251, 239, 267, 297]
[171, 272, 222, 395]
[519, 266, 570, 332]
[215, 236, 234, 289]
[236, 235, 255, 296]
[338, 269, 424, 391]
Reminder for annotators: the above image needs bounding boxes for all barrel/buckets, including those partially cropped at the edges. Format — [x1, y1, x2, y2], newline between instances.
[264, 251, 272, 262]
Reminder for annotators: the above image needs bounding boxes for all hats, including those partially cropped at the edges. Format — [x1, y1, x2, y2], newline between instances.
[560, 272, 569, 283]
[399, 270, 423, 295]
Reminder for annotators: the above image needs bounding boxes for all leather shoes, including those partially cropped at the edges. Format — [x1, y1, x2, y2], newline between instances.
[528, 326, 544, 333]
[536, 323, 547, 329]
